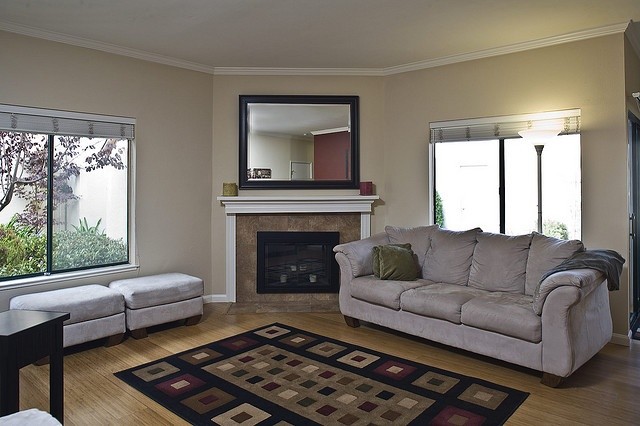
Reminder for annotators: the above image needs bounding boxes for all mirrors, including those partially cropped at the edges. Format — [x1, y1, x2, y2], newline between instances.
[238, 94, 360, 189]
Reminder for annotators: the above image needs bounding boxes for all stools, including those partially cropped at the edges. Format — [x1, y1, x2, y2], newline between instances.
[8, 283, 126, 365]
[109, 271, 204, 339]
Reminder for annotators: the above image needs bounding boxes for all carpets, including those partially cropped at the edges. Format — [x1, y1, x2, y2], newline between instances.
[113, 322, 532, 426]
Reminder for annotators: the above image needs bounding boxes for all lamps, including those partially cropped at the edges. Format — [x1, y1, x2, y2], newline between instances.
[517, 123, 567, 234]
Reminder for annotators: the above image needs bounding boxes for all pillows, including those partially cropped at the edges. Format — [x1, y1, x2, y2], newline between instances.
[379, 242, 416, 280]
[372, 245, 418, 279]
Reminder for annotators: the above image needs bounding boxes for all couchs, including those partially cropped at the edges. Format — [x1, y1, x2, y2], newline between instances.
[332, 225, 625, 388]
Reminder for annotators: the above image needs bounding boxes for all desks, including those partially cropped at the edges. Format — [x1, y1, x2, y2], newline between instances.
[0, 311, 70, 426]
